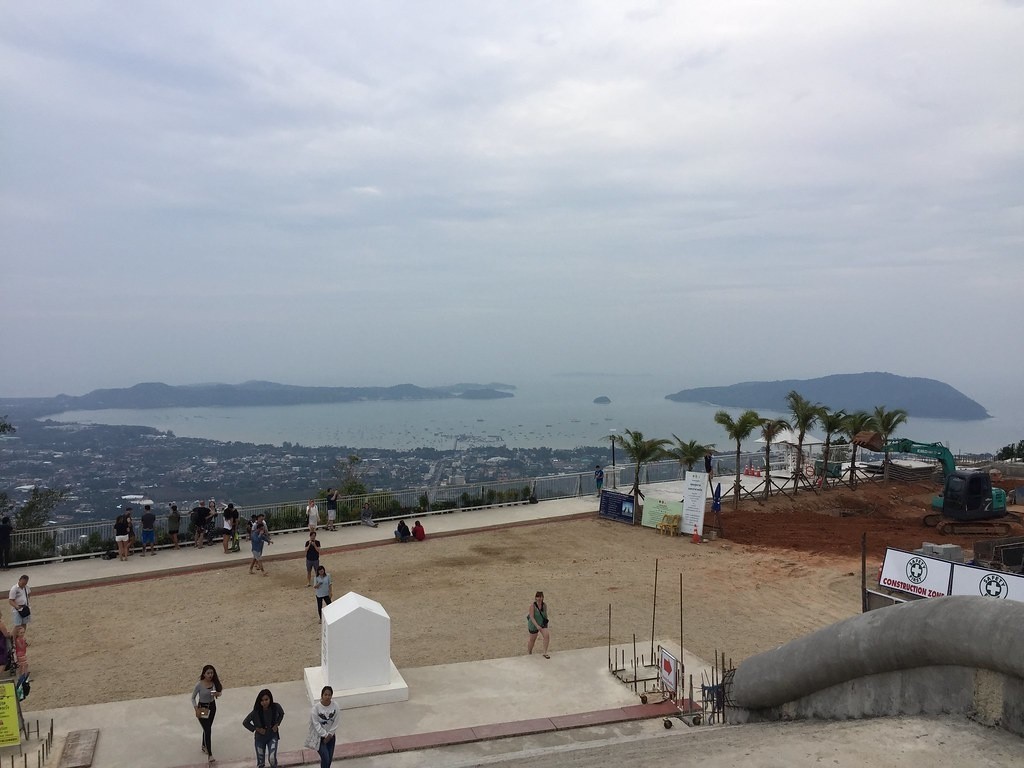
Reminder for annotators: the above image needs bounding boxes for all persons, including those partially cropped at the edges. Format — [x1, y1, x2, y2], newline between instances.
[306, 499, 320, 530]
[361, 503, 379, 526]
[0, 574, 31, 681]
[325, 488, 341, 531]
[246, 514, 273, 576]
[310, 686, 341, 768]
[168, 506, 181, 550]
[0, 517, 13, 571]
[394, 520, 410, 543]
[528, 592, 551, 659]
[304, 532, 321, 587]
[139, 505, 157, 557]
[114, 507, 135, 560]
[186, 501, 218, 548]
[411, 521, 426, 541]
[704, 453, 713, 479]
[223, 503, 240, 554]
[313, 566, 333, 624]
[191, 665, 222, 762]
[242, 688, 284, 768]
[593, 465, 605, 497]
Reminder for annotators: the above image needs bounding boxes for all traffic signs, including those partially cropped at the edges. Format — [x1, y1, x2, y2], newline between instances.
[660, 649, 677, 692]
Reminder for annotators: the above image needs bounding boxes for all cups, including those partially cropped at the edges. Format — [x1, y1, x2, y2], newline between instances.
[210, 690, 216, 696]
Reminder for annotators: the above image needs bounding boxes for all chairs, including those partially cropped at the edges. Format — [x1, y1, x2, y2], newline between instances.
[656, 514, 682, 536]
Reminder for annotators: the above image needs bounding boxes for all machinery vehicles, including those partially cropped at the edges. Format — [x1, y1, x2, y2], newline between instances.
[849, 431, 1023, 537]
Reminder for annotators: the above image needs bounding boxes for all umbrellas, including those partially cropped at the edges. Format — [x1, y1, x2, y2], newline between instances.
[755, 427, 824, 475]
[711, 482, 721, 530]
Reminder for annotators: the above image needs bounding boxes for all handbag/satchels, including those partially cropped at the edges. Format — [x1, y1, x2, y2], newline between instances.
[18, 606, 30, 617]
[543, 618, 549, 625]
[304, 705, 320, 751]
[196, 708, 210, 719]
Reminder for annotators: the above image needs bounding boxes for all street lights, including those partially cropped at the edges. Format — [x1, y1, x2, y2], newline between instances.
[610, 435, 618, 490]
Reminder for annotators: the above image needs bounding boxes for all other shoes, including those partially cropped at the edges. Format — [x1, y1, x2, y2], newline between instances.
[201, 747, 208, 754]
[208, 756, 215, 762]
[543, 654, 550, 659]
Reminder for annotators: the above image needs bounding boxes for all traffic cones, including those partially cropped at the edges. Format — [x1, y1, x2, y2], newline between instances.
[749, 464, 755, 476]
[818, 476, 822, 487]
[691, 523, 702, 543]
[743, 464, 749, 476]
[878, 558, 884, 582]
[755, 466, 762, 477]
